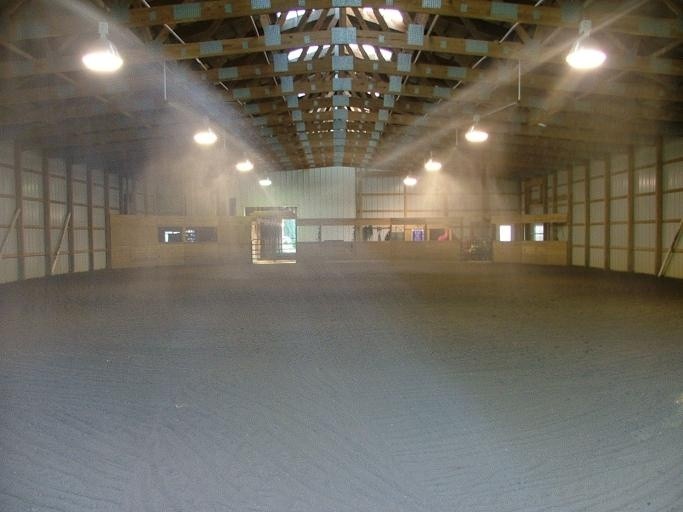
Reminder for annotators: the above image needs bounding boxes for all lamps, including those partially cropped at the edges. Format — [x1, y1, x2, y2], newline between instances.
[423, 150, 441, 173]
[258, 167, 274, 186]
[464, 113, 489, 143]
[402, 168, 419, 187]
[234, 152, 255, 172]
[192, 116, 219, 146]
[565, 19, 606, 71]
[81, 21, 123, 73]
